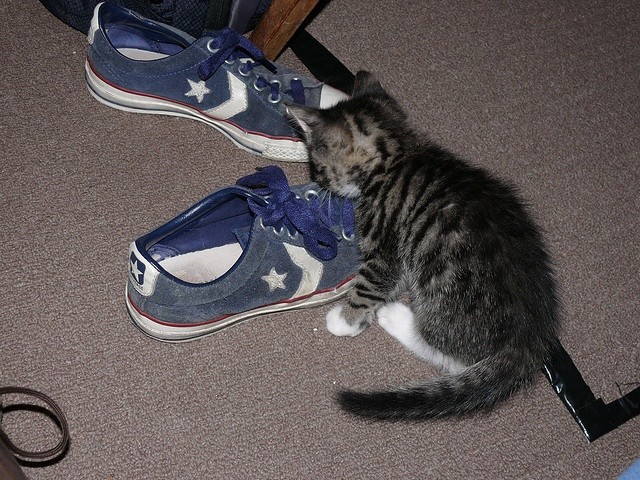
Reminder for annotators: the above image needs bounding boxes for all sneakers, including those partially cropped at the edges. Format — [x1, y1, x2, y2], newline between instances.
[84, 2, 351, 163]
[124, 165, 367, 344]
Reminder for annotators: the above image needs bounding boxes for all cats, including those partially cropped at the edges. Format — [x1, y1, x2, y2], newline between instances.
[279, 68, 566, 428]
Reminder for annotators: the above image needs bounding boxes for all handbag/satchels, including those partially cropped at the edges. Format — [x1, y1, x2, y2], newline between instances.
[39, 1, 356, 95]
